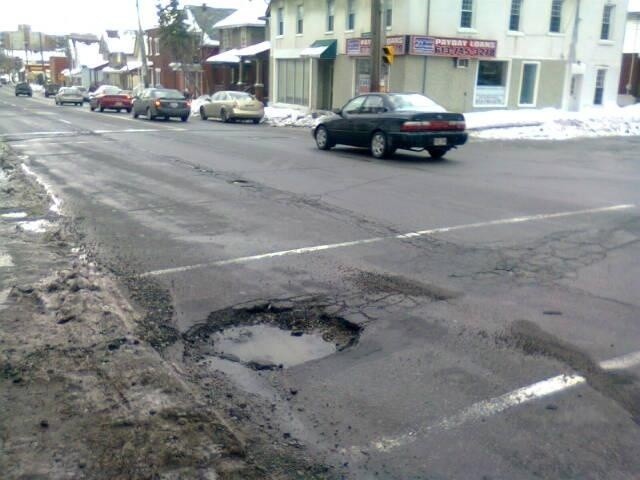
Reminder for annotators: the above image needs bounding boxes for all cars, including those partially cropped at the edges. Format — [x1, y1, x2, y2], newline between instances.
[199, 90, 265, 123]
[14, 80, 212, 122]
[310, 91, 469, 162]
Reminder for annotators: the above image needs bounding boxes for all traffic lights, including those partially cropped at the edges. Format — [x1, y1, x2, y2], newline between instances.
[381, 45, 395, 66]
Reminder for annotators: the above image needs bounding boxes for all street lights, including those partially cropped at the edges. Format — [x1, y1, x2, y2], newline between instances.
[23, 39, 30, 85]
